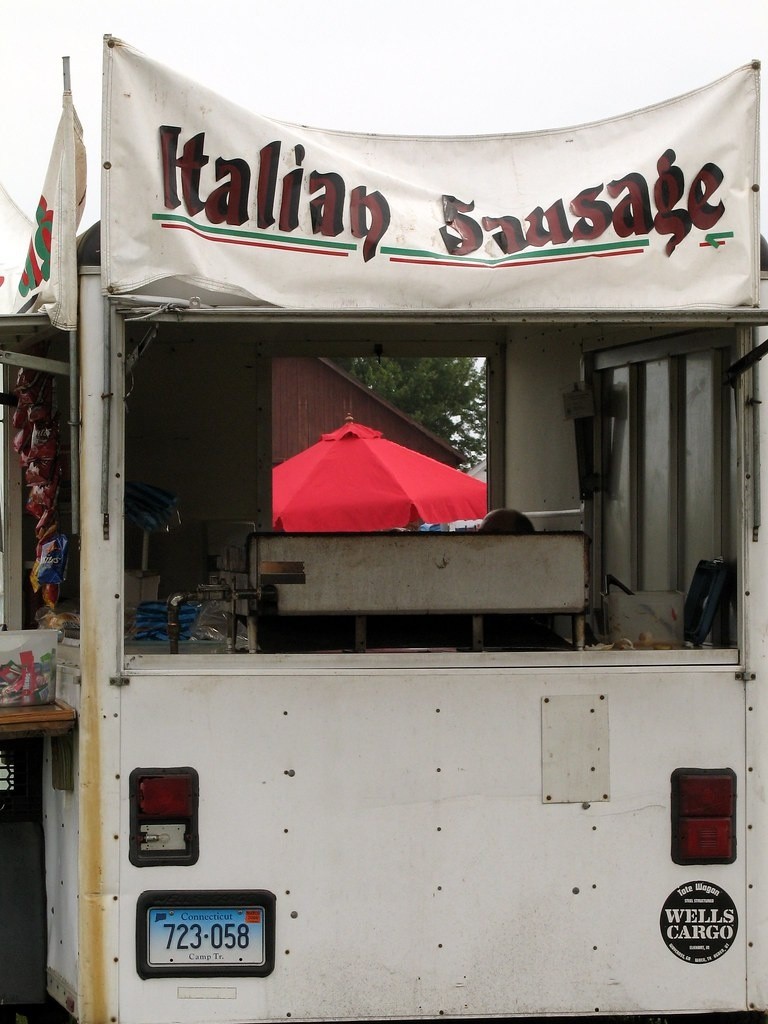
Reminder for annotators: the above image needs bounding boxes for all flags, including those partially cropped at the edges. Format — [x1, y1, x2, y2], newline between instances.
[8, 102, 89, 331]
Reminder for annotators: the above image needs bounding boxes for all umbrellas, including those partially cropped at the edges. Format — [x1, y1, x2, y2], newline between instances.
[272, 412, 488, 531]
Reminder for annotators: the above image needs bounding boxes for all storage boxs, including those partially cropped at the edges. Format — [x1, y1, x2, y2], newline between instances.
[608, 590, 686, 649]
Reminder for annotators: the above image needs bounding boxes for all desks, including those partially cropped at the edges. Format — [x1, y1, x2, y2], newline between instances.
[0, 698, 77, 740]
